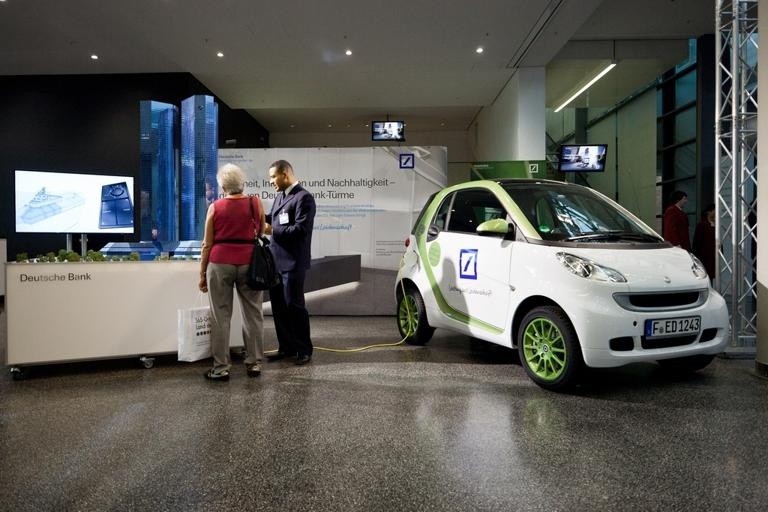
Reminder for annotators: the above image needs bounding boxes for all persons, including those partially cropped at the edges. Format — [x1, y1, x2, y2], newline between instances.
[663, 190, 691, 252]
[264, 160, 316, 365]
[198, 161, 266, 383]
[692, 203, 716, 287]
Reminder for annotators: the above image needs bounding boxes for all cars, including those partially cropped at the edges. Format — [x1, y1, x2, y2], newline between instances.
[394, 177, 730, 388]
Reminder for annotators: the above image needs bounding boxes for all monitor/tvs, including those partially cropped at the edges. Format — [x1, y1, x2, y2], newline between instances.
[558, 144, 608, 173]
[371, 121, 404, 141]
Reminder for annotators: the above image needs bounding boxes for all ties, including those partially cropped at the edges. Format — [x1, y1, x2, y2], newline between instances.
[280, 191, 285, 203]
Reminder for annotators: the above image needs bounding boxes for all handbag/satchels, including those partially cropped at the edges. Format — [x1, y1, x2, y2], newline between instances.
[246, 246, 280, 291]
[178, 306, 212, 363]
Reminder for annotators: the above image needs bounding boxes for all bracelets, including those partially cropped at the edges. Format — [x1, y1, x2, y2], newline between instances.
[200, 271, 205, 276]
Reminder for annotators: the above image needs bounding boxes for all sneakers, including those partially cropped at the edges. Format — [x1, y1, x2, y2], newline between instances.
[247, 364, 260, 375]
[205, 368, 230, 381]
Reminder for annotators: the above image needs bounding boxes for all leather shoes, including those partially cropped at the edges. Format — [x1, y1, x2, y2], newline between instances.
[296, 351, 310, 365]
[266, 351, 297, 359]
